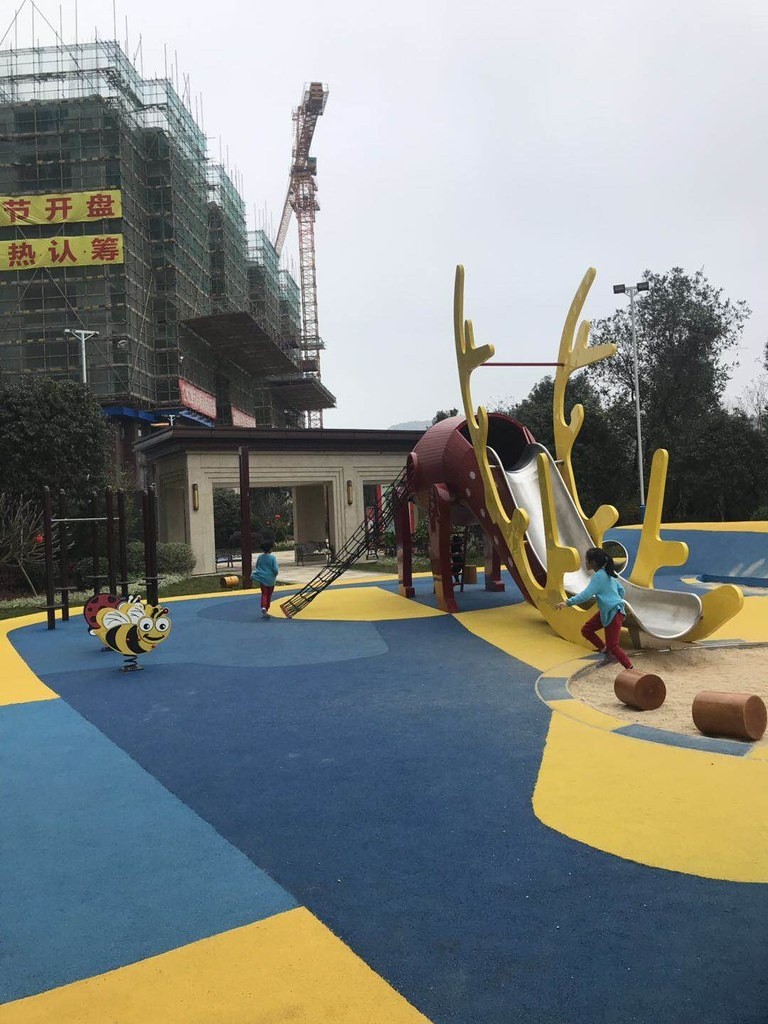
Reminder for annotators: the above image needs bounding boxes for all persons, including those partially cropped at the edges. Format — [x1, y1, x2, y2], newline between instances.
[553, 548, 635, 671]
[450, 548, 464, 584]
[367, 516, 374, 546]
[250, 541, 278, 618]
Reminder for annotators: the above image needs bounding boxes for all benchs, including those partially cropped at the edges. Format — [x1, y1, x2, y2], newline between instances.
[295, 541, 335, 566]
[366, 532, 395, 560]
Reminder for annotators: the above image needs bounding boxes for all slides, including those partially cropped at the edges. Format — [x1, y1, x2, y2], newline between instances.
[486, 443, 702, 640]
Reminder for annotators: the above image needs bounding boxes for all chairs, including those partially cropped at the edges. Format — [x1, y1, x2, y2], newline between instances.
[230, 547, 242, 567]
[215, 549, 230, 568]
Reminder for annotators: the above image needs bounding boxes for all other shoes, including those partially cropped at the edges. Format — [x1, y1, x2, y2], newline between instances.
[261, 608, 268, 618]
